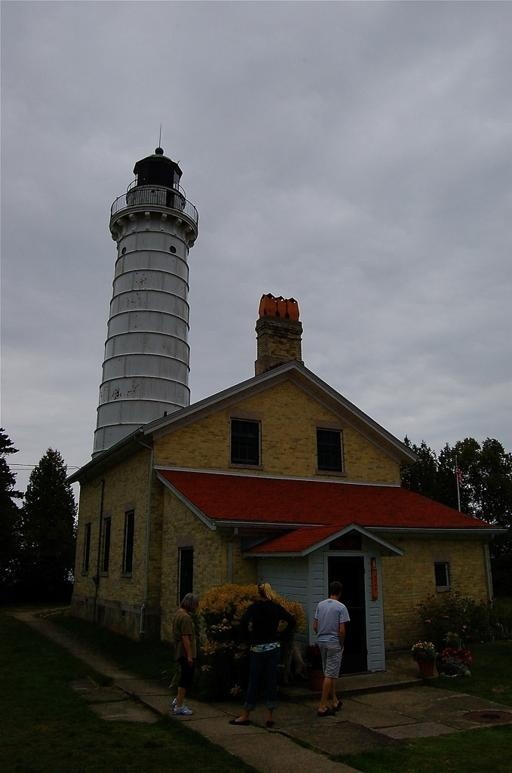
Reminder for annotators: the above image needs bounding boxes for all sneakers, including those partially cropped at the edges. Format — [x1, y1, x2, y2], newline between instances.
[266, 720, 274, 727]
[229, 719, 250, 726]
[172, 699, 192, 716]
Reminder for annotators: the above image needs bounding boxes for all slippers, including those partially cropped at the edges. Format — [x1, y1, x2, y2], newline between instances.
[335, 701, 343, 710]
[317, 706, 335, 717]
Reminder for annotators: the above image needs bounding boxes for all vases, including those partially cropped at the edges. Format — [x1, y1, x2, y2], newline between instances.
[414, 652, 436, 676]
[436, 654, 458, 680]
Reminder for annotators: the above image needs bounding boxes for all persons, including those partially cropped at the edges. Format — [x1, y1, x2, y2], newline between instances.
[229, 581, 298, 727]
[171, 592, 201, 716]
[311, 580, 352, 716]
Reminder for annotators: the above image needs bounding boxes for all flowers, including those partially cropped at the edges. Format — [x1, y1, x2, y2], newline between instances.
[441, 646, 473, 676]
[411, 640, 440, 663]
